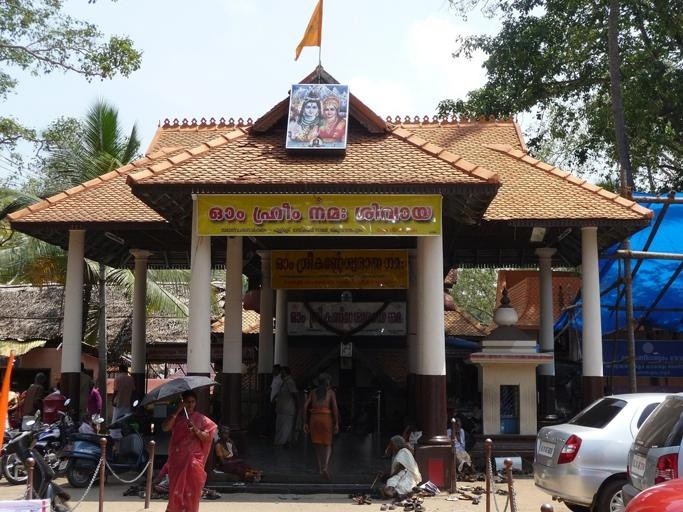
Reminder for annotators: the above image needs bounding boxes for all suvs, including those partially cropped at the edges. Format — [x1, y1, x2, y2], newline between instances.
[621, 392, 682, 507]
[531, 393, 671, 512]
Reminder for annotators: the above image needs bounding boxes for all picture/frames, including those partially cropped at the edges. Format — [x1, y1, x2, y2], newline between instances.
[284, 84, 349, 150]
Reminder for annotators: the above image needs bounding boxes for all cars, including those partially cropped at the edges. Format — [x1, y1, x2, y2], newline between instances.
[622, 478, 683, 512]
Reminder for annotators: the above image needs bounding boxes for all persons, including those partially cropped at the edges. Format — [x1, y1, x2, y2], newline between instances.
[266, 362, 282, 403]
[300, 371, 340, 480]
[111, 364, 139, 426]
[310, 95, 345, 143]
[447, 415, 476, 481]
[554, 374, 617, 416]
[649, 376, 664, 392]
[661, 378, 671, 392]
[377, 433, 423, 499]
[11, 372, 60, 427]
[287, 92, 324, 142]
[211, 416, 263, 483]
[80, 363, 102, 425]
[271, 365, 301, 450]
[161, 390, 217, 512]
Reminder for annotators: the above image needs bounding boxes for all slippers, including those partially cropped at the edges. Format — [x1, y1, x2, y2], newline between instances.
[404, 501, 414, 511]
[413, 502, 424, 512]
[122, 475, 221, 499]
[351, 494, 372, 504]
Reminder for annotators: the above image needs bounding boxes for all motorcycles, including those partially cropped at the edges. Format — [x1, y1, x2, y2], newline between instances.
[56, 399, 149, 488]
[0, 399, 101, 483]
[0, 410, 71, 512]
